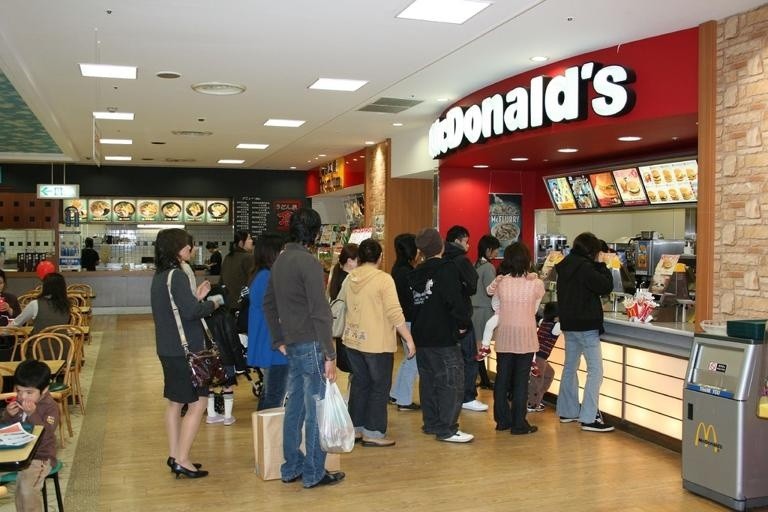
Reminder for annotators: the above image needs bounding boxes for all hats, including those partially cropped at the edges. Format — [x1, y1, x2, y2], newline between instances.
[416, 228, 442, 258]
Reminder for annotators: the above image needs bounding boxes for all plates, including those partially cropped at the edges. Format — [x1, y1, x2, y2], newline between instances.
[698, 318, 727, 335]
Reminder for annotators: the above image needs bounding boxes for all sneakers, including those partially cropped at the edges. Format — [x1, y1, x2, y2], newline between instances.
[560, 416, 578, 423]
[206, 414, 224, 424]
[223, 416, 236, 425]
[462, 399, 489, 411]
[530, 361, 543, 377]
[390, 397, 397, 404]
[527, 402, 545, 412]
[511, 426, 537, 435]
[581, 413, 615, 432]
[478, 383, 496, 391]
[355, 432, 363, 442]
[398, 402, 420, 411]
[362, 435, 395, 447]
[474, 346, 491, 362]
[442, 430, 474, 443]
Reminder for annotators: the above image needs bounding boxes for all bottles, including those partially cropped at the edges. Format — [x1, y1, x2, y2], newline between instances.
[61, 247, 79, 256]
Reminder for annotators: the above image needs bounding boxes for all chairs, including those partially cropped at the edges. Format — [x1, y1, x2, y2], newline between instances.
[0, 326, 28, 362]
[16, 285, 42, 326]
[0, 391, 64, 512]
[66, 283, 92, 325]
[37, 325, 84, 416]
[13, 333, 75, 449]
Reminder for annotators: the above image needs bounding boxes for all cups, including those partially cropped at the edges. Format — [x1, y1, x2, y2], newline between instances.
[639, 303, 655, 322]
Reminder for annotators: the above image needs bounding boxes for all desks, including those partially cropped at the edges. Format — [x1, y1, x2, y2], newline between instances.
[0, 360, 66, 393]
[0, 425, 45, 471]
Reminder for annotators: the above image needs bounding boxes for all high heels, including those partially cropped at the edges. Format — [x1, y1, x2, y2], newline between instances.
[172, 463, 208, 479]
[167, 456, 202, 473]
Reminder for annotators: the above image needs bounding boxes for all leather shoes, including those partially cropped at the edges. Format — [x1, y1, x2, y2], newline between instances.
[303, 472, 344, 489]
[282, 474, 303, 483]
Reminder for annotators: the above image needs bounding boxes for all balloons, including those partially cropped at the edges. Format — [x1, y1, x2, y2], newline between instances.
[36, 261, 56, 282]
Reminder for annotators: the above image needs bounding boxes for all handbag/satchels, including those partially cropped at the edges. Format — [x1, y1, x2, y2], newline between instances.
[236, 295, 249, 331]
[330, 298, 347, 338]
[184, 342, 230, 388]
[251, 405, 342, 481]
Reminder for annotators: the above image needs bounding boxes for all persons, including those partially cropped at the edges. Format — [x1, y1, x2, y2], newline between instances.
[151, 228, 247, 479]
[10, 273, 72, 360]
[527, 232, 615, 431]
[0, 361, 60, 512]
[207, 209, 345, 487]
[0, 269, 22, 319]
[327, 224, 545, 447]
[81, 237, 101, 271]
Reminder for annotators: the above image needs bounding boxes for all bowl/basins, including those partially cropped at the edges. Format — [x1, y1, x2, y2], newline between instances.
[208, 203, 228, 219]
[89, 201, 111, 218]
[491, 222, 519, 249]
[162, 202, 180, 219]
[138, 202, 159, 218]
[114, 202, 135, 219]
[186, 202, 204, 219]
